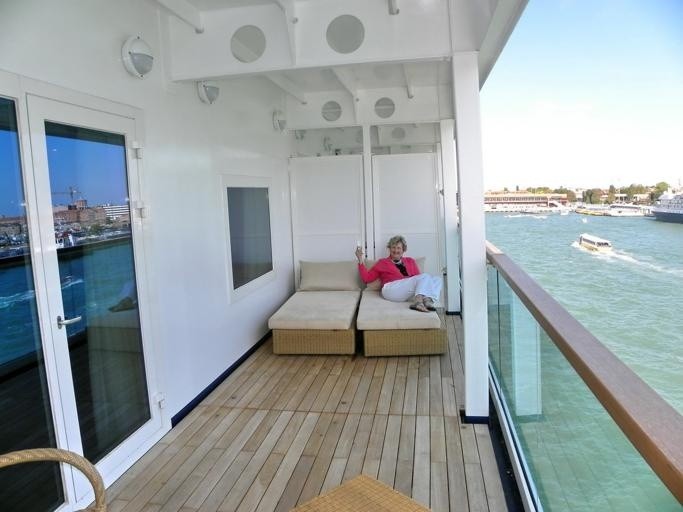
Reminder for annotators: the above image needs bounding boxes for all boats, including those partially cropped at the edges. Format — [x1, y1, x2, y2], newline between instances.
[575, 204, 654, 217]
[579, 233, 612, 253]
[30, 276, 71, 294]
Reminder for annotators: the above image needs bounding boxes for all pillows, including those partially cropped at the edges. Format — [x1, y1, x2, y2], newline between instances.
[296, 260, 361, 291]
[364, 257, 426, 291]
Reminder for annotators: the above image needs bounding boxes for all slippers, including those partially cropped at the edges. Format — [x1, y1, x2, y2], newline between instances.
[423, 300, 436, 311]
[410, 302, 428, 312]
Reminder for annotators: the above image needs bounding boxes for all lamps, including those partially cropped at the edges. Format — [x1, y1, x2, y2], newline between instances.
[274, 109, 287, 133]
[198, 80, 220, 106]
[122, 35, 155, 78]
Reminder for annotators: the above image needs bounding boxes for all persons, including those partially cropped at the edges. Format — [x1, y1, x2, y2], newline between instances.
[354, 235, 443, 313]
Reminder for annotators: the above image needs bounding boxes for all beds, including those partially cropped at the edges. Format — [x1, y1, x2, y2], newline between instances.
[267, 260, 362, 355]
[86, 300, 143, 353]
[357, 256, 449, 357]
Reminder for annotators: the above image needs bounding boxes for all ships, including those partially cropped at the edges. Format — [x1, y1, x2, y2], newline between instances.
[651, 195, 683, 223]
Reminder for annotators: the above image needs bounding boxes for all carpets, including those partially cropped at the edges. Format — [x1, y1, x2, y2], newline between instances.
[286, 472, 435, 512]
